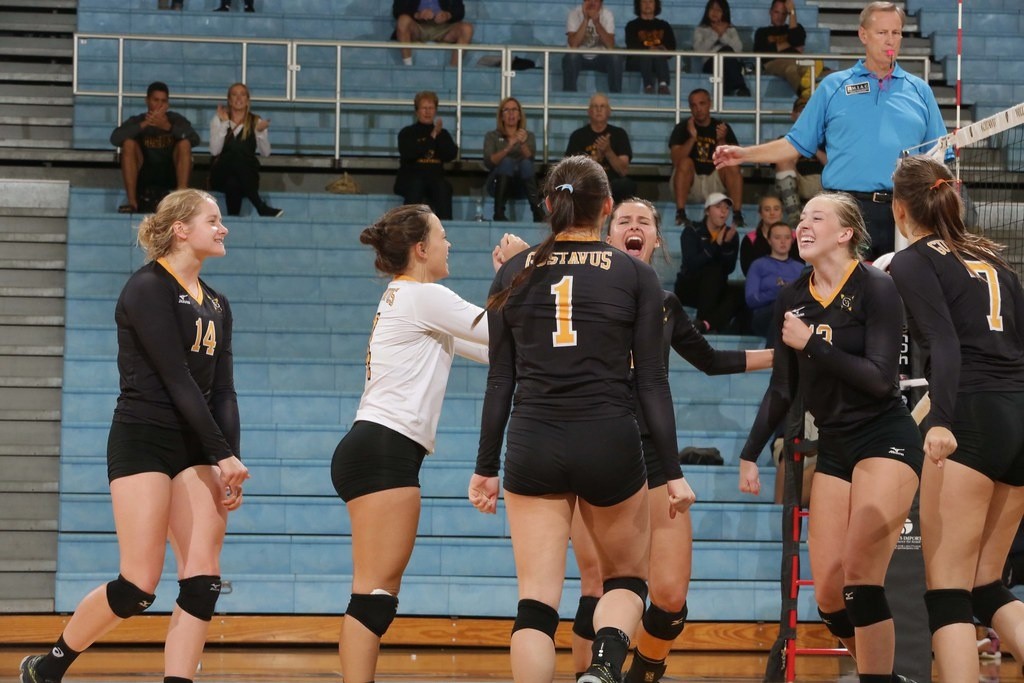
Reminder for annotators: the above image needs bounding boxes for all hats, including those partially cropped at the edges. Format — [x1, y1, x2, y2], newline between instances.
[704, 192, 733, 208]
[793, 97, 808, 107]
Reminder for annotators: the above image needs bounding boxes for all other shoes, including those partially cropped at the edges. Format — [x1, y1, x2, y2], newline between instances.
[578, 663, 621, 683]
[244, 6, 256, 12]
[725, 89, 752, 97]
[801, 60, 824, 88]
[801, 83, 819, 97]
[214, 5, 230, 12]
[659, 86, 671, 94]
[646, 86, 656, 94]
[732, 217, 749, 227]
[675, 212, 693, 226]
[258, 202, 284, 217]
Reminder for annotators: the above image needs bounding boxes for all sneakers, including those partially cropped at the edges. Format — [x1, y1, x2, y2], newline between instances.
[19, 654, 62, 683]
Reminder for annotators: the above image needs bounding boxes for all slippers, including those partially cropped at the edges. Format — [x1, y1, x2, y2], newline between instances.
[119, 205, 143, 213]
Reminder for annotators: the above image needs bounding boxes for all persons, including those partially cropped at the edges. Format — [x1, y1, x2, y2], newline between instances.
[331, 205, 530, 682]
[674, 192, 807, 349]
[468, 156, 694, 683]
[565, 92, 632, 205]
[571, 197, 774, 683]
[693, 0, 823, 99]
[482, 98, 545, 222]
[394, 91, 460, 220]
[561, 0, 677, 95]
[391, 0, 473, 66]
[19, 189, 250, 683]
[770, 252, 1024, 659]
[208, 83, 283, 217]
[110, 81, 201, 215]
[739, 193, 924, 683]
[770, 95, 827, 227]
[712, 2, 955, 262]
[158, 0, 255, 13]
[889, 159, 1024, 683]
[669, 89, 748, 229]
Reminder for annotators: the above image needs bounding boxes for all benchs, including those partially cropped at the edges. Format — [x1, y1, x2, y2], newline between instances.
[56, 0, 1024, 622]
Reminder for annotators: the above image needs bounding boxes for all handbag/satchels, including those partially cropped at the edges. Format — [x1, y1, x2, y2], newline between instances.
[681, 446, 725, 466]
[326, 172, 364, 194]
[201, 171, 213, 190]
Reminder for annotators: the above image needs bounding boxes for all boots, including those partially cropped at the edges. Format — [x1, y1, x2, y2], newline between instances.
[521, 176, 545, 222]
[493, 173, 510, 221]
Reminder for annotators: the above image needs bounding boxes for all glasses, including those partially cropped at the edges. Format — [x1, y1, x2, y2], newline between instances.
[503, 107, 520, 113]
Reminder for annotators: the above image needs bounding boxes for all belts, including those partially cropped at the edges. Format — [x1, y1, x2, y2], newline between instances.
[829, 189, 893, 203]
